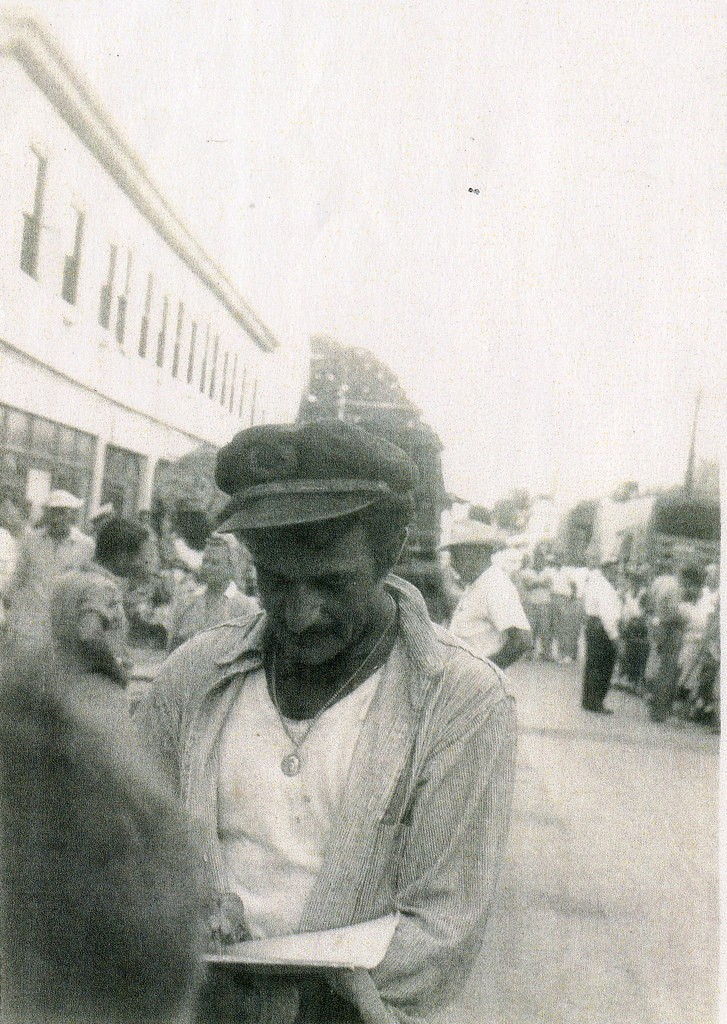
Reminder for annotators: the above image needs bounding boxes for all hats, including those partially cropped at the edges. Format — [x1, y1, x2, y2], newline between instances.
[600, 555, 621, 568]
[215, 417, 422, 534]
[40, 488, 84, 509]
[435, 518, 506, 553]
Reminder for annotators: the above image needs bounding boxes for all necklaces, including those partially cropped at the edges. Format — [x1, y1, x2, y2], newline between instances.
[271, 591, 398, 778]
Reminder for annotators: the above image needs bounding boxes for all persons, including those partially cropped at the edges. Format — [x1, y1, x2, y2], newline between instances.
[522, 547, 720, 730]
[1, 427, 533, 1023]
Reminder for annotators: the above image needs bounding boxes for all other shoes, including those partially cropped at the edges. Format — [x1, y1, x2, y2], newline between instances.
[589, 705, 616, 715]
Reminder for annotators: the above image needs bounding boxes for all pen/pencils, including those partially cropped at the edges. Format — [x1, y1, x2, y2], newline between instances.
[201, 849, 225, 958]
